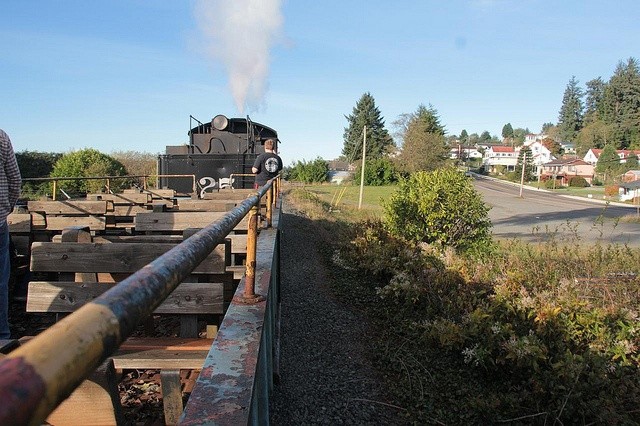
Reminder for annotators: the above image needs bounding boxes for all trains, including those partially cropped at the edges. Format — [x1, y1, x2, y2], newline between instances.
[0, 115, 283, 426]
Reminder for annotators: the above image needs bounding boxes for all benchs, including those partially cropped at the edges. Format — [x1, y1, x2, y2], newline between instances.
[6, 188, 263, 294]
[27, 238, 226, 426]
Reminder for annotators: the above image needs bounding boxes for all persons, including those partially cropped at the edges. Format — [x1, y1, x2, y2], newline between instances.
[251, 139, 283, 190]
[2, 129, 22, 339]
